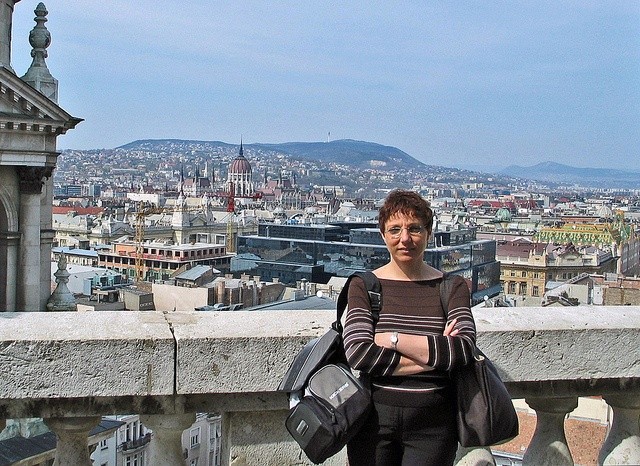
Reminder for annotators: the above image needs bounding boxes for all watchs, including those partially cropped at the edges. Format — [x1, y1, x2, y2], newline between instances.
[389, 328, 401, 352]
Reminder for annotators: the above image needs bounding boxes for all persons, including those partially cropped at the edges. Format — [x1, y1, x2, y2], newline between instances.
[341, 187, 479, 466]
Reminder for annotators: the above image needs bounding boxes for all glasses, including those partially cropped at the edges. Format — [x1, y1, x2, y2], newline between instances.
[384, 224, 426, 236]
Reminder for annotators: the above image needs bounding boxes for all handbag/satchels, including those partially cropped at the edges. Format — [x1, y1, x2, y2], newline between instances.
[277, 271, 381, 464]
[439, 273, 519, 448]
